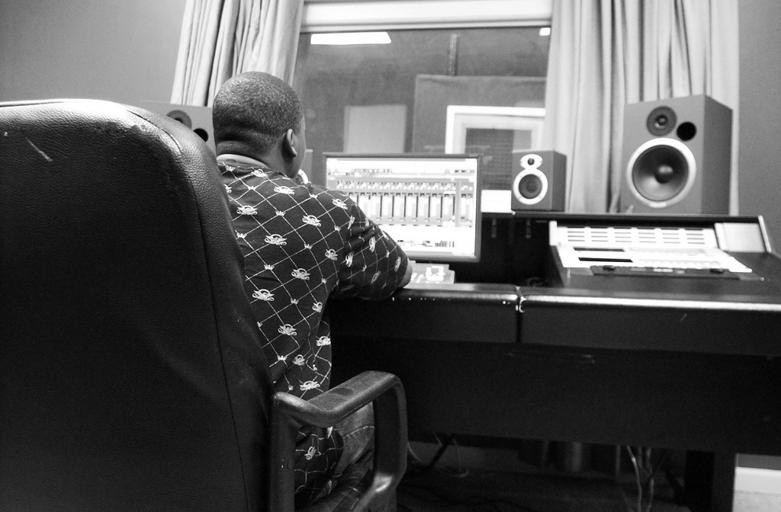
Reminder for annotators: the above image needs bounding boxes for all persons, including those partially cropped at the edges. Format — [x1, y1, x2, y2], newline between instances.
[206, 72, 414, 508]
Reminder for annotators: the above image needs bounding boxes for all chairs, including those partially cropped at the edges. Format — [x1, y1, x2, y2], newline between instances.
[3, 100, 407, 512]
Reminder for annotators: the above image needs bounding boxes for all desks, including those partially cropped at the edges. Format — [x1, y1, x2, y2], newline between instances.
[324, 248, 780, 511]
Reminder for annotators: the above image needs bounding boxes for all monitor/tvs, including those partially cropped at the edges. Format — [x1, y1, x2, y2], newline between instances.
[321, 151, 483, 264]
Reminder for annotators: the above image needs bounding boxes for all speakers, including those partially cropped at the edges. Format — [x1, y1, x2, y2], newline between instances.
[511, 150, 566, 211]
[620, 94, 733, 214]
[139, 103, 217, 157]
